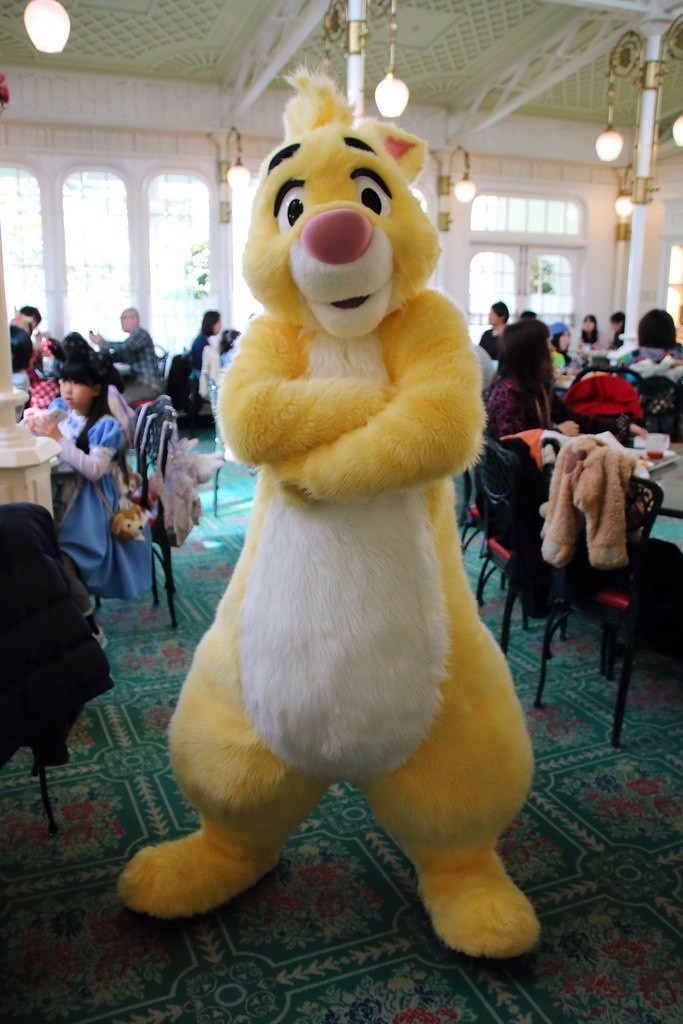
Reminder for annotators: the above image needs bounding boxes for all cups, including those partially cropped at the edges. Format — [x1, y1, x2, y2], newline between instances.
[646, 433, 668, 460]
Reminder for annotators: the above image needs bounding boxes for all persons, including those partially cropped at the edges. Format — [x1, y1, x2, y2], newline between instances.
[0, 307, 166, 775]
[185, 312, 258, 414]
[478, 302, 683, 660]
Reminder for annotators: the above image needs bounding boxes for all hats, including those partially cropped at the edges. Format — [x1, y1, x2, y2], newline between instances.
[550, 324, 568, 341]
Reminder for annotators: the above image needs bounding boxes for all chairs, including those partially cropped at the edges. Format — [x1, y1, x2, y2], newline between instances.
[477, 437, 571, 640]
[532, 478, 664, 746]
[94, 394, 181, 628]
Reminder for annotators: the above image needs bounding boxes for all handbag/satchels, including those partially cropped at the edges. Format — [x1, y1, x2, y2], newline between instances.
[111, 503, 148, 543]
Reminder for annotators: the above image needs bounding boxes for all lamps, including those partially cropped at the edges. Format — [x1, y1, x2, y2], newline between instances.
[450, 146, 478, 204]
[613, 163, 634, 218]
[22, 0, 70, 53]
[596, 72, 624, 164]
[672, 93, 683, 148]
[375, 0, 409, 117]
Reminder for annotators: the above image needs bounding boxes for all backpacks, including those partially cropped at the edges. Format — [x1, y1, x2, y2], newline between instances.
[134, 402, 221, 548]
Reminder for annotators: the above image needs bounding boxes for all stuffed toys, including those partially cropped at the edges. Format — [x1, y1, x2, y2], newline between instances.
[110, 504, 146, 543]
[118, 70, 539, 958]
